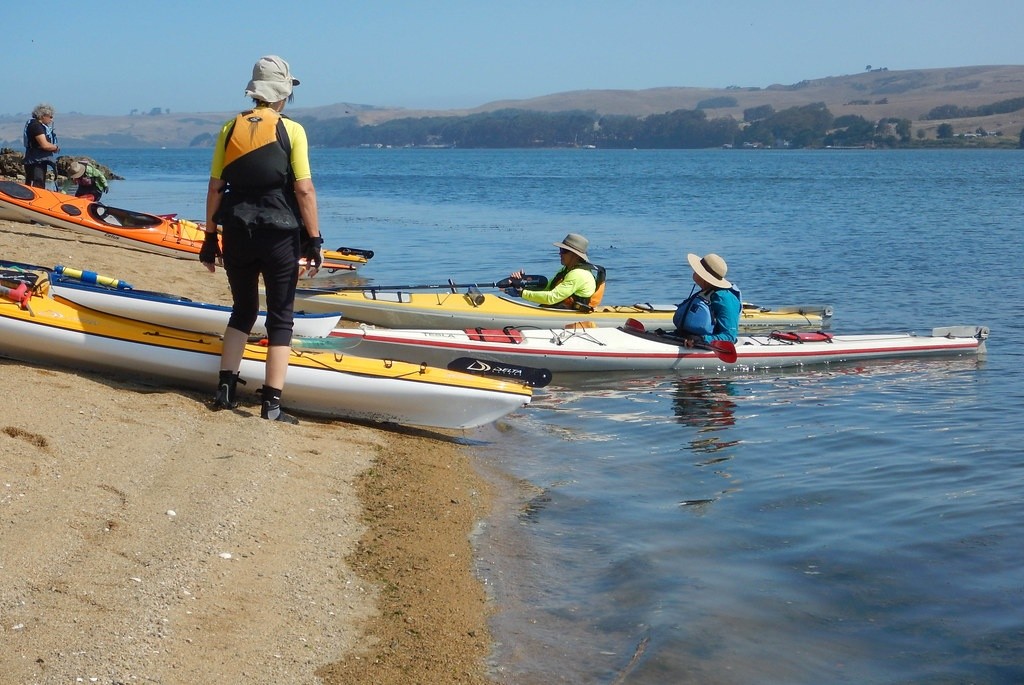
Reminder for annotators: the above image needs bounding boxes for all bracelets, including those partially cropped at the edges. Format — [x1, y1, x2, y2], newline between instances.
[57, 146, 58, 148]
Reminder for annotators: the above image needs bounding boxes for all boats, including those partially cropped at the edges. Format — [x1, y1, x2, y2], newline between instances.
[0, 257, 342, 341]
[326, 324, 990, 373]
[225, 281, 832, 334]
[0, 267, 552, 431]
[1, 178, 375, 282]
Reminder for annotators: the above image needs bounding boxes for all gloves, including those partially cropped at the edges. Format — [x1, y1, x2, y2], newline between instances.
[102, 187, 109, 194]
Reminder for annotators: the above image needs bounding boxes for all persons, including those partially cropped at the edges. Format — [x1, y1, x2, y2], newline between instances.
[24, 105, 60, 188]
[655, 253, 742, 348]
[499, 234, 596, 307]
[199, 56, 323, 424]
[62, 162, 109, 202]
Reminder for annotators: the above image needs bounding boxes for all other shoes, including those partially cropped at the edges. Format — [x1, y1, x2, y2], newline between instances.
[213, 382, 239, 411]
[263, 399, 299, 425]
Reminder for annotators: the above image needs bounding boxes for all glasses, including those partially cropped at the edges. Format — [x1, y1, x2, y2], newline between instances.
[559, 247, 570, 254]
[45, 116, 53, 120]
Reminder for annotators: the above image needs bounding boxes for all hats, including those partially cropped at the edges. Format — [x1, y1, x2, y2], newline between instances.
[67, 163, 85, 179]
[687, 253, 732, 288]
[553, 233, 590, 263]
[245, 56, 299, 103]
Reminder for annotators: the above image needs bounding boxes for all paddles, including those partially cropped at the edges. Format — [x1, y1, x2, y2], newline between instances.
[308, 274, 550, 297]
[624, 319, 738, 363]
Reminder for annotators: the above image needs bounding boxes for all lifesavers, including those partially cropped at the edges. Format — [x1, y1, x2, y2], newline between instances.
[54, 264, 132, 291]
